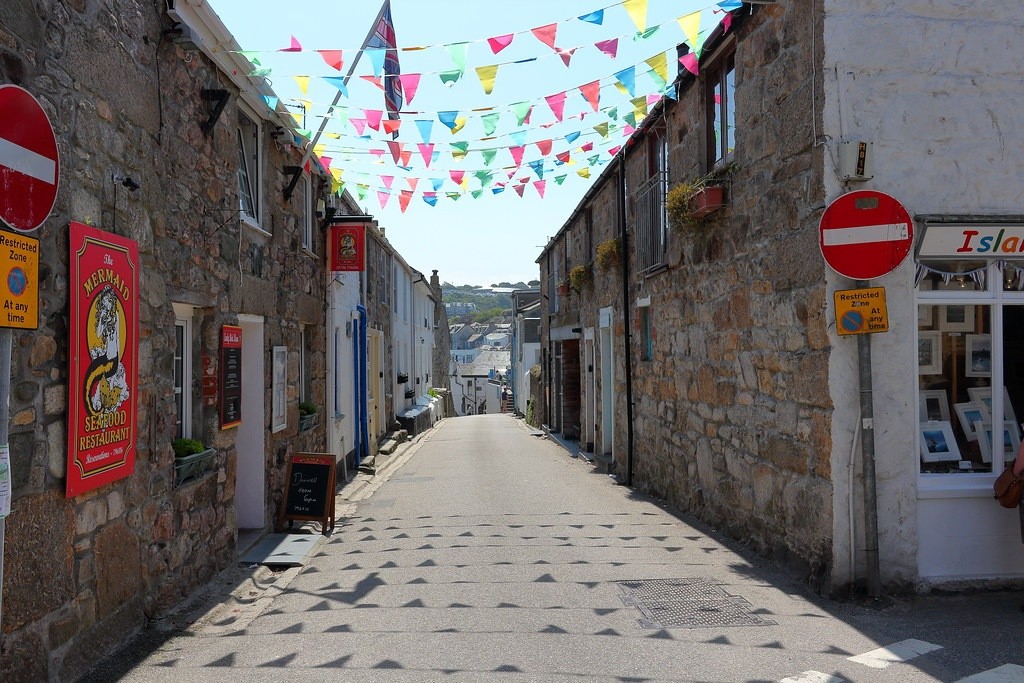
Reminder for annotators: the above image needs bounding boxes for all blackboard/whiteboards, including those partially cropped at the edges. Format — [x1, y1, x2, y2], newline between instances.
[278, 452, 336, 521]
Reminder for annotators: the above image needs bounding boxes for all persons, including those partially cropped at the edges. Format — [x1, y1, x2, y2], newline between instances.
[494, 370, 502, 381]
[502, 388, 509, 414]
[1013, 438, 1024, 544]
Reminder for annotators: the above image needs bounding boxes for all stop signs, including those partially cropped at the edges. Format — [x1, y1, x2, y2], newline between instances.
[818, 189, 915, 281]
[0, 84, 61, 231]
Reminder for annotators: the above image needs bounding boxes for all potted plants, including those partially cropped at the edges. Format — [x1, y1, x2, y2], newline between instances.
[555, 264, 593, 297]
[595, 236, 623, 273]
[663, 162, 730, 232]
[172, 438, 218, 482]
[298, 403, 319, 430]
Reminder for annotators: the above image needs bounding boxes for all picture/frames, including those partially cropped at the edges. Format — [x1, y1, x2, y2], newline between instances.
[917, 277, 1022, 463]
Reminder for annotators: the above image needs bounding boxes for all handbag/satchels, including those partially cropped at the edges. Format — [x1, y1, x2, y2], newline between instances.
[992, 458, 1024, 508]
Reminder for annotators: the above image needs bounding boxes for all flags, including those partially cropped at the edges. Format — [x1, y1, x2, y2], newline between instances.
[364, 1, 402, 140]
